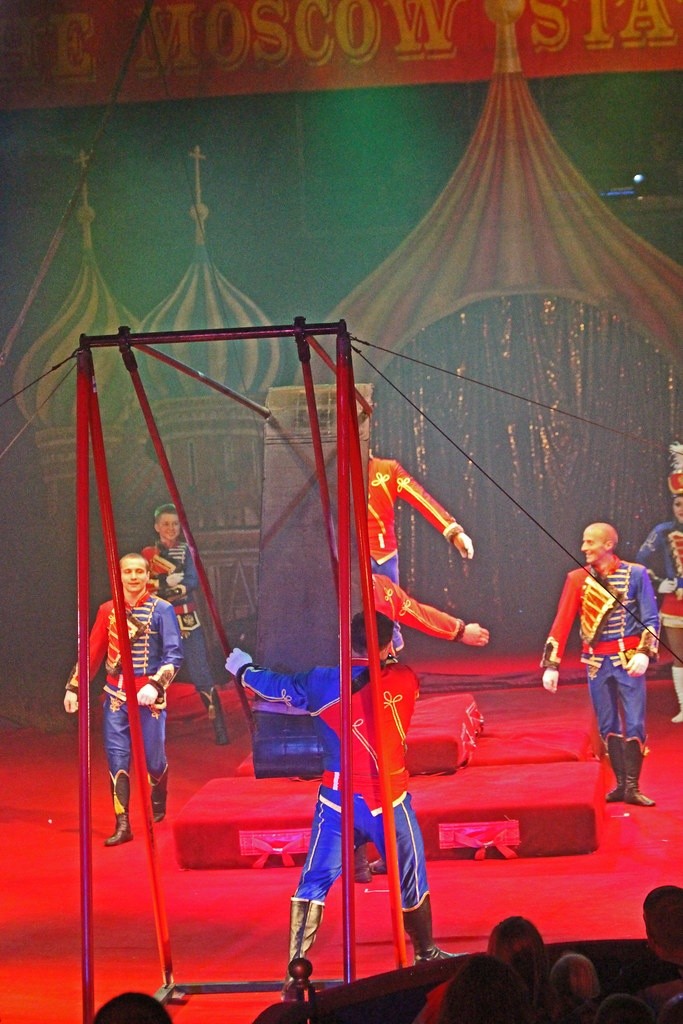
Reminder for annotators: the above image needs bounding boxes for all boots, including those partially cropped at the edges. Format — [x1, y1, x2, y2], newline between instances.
[282, 895, 324, 1001]
[624, 734, 656, 805]
[104, 768, 133, 846]
[669, 666, 682, 722]
[201, 686, 230, 745]
[149, 763, 171, 823]
[401, 891, 470, 965]
[371, 857, 386, 875]
[354, 843, 373, 883]
[599, 732, 627, 802]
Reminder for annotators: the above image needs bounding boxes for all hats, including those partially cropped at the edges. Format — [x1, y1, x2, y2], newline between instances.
[667, 440, 683, 497]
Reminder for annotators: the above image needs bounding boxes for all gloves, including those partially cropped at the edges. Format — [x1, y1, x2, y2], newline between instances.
[452, 532, 474, 559]
[166, 572, 185, 587]
[542, 669, 560, 693]
[224, 648, 254, 676]
[625, 652, 650, 678]
[137, 684, 159, 706]
[64, 691, 78, 713]
[657, 577, 678, 594]
[174, 585, 186, 597]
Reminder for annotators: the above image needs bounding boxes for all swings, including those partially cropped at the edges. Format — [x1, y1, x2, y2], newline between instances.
[91, 335, 341, 779]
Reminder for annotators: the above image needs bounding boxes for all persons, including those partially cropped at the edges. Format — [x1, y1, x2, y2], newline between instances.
[614, 885, 683, 993]
[140, 503, 230, 746]
[92, 992, 173, 1024]
[411, 916, 683, 1024]
[539, 523, 661, 806]
[371, 574, 490, 665]
[367, 449, 475, 664]
[224, 610, 469, 1000]
[63, 553, 186, 847]
[636, 489, 683, 723]
